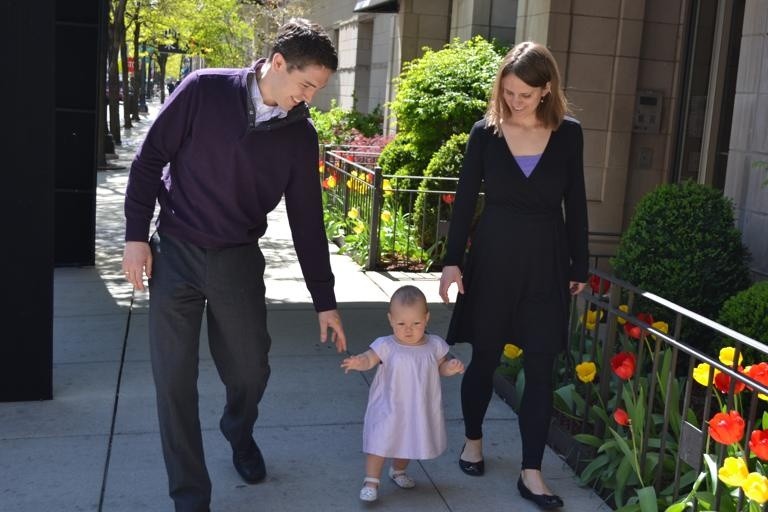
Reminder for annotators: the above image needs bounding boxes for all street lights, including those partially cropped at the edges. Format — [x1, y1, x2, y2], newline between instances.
[146, 47, 154, 98]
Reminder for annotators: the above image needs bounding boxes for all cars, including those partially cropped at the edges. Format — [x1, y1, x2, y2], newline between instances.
[105, 79, 128, 104]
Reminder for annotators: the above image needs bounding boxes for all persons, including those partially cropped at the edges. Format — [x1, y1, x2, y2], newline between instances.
[340, 285, 469, 502]
[166, 78, 175, 95]
[124, 17, 350, 511]
[439, 38, 593, 511]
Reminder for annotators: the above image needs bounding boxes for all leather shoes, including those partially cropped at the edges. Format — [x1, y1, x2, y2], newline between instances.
[219, 417, 267, 484]
[517, 471, 565, 510]
[359, 477, 381, 501]
[457, 443, 486, 476]
[387, 465, 416, 489]
